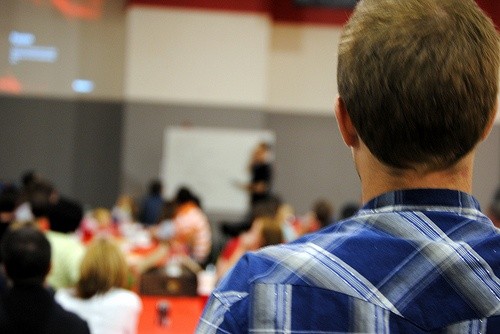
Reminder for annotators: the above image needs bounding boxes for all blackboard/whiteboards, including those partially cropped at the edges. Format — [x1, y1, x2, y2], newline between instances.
[159, 125, 274, 222]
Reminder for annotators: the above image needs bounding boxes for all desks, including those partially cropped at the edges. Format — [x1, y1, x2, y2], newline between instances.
[136, 294, 209, 334]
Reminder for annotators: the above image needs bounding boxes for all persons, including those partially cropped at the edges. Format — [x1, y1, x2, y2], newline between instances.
[193, 0, 500, 334]
[0, 169, 500, 334]
[242, 142, 274, 214]
